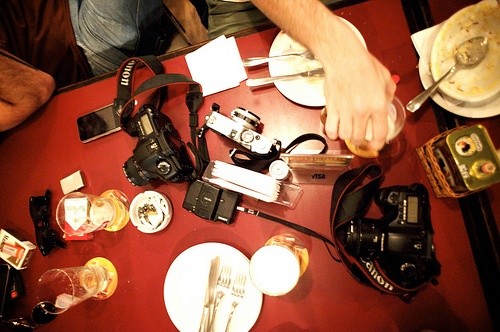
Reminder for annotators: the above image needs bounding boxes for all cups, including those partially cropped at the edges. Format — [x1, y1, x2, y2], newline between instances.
[341, 91, 406, 159]
[248, 233, 310, 297]
[55, 188, 130, 236]
[36, 257, 118, 315]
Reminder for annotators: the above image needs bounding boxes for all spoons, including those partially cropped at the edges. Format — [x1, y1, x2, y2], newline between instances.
[241, 49, 316, 67]
[406, 36, 488, 112]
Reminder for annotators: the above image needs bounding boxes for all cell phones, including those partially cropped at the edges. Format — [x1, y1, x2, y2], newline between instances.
[76, 103, 121, 143]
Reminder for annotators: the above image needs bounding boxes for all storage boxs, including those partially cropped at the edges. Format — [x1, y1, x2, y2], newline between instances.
[59, 170, 83, 194]
[63, 197, 93, 240]
[0, 227, 37, 270]
[425, 124, 500, 193]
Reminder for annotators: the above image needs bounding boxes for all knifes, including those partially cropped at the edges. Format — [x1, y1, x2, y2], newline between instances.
[245, 68, 324, 87]
[199, 255, 220, 332]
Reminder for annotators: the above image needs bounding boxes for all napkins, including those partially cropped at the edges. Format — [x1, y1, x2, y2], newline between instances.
[184, 34, 248, 96]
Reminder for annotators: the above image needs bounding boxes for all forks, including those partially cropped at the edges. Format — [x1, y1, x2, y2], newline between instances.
[210, 265, 246, 332]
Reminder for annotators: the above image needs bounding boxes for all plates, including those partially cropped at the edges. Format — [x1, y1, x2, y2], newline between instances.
[267, 15, 367, 107]
[430, 4, 500, 103]
[163, 242, 263, 332]
[418, 19, 500, 119]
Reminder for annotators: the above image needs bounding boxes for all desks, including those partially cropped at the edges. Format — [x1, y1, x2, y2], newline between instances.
[0, 0, 500, 332]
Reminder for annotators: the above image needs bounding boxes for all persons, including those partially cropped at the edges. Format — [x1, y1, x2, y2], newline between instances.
[0, 0, 166, 132]
[206, 0, 400, 153]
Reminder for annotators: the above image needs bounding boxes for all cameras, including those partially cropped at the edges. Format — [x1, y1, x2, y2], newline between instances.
[183, 180, 239, 224]
[337, 182, 441, 288]
[122, 105, 194, 187]
[207, 107, 273, 155]
